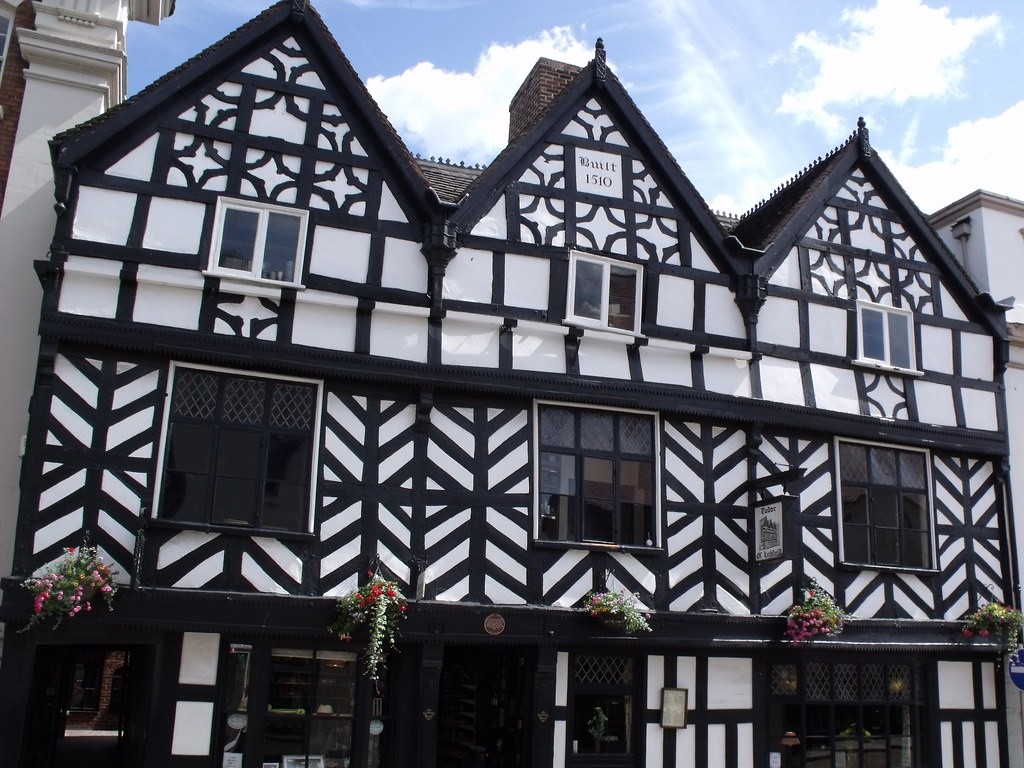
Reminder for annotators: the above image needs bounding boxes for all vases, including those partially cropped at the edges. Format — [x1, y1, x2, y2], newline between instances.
[78, 582, 97, 602]
[599, 611, 625, 630]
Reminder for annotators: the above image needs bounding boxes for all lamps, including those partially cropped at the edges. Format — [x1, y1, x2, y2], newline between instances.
[780, 731, 801, 768]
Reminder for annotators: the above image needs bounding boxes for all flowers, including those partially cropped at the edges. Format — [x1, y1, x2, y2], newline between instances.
[963, 601, 1024, 671]
[336, 570, 409, 680]
[787, 592, 848, 647]
[15, 547, 120, 635]
[583, 590, 654, 634]
